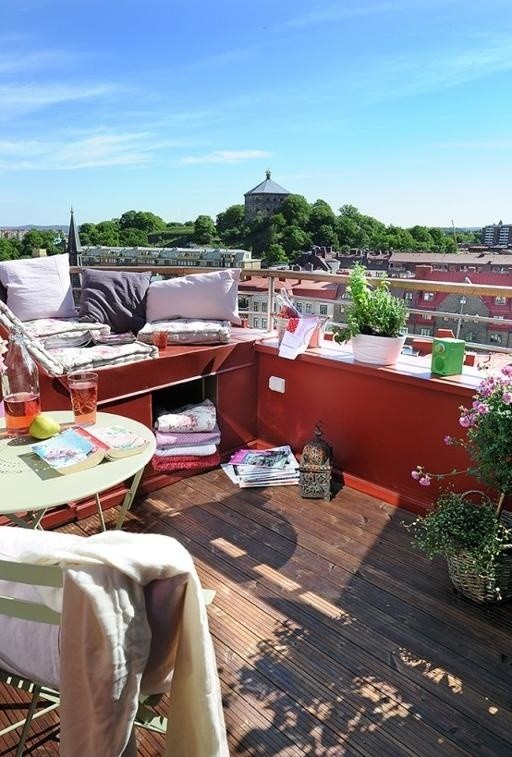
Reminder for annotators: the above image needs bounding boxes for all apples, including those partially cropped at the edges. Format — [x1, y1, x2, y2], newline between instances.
[435, 343, 445, 354]
[434, 357, 444, 371]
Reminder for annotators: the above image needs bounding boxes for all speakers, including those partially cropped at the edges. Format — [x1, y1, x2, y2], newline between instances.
[431, 338, 466, 375]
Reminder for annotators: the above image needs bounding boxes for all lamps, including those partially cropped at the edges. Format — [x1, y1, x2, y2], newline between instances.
[294, 419, 334, 501]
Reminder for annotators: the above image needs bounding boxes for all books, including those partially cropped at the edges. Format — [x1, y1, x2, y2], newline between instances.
[30, 419, 153, 476]
[219, 442, 301, 489]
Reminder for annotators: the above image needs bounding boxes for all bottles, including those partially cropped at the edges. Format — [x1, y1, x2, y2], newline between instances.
[0, 323, 42, 435]
[276, 287, 302, 318]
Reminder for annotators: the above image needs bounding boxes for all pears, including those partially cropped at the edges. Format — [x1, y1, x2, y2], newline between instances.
[29, 416, 59, 439]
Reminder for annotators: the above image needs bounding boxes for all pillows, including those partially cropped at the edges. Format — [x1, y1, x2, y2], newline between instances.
[77, 265, 152, 336]
[0, 561, 190, 696]
[146, 267, 242, 326]
[0, 252, 79, 322]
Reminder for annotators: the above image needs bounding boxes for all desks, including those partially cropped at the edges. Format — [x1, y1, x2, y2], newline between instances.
[0, 412, 157, 531]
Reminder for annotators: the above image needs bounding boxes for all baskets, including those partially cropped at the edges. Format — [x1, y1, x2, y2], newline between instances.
[446, 508, 512, 603]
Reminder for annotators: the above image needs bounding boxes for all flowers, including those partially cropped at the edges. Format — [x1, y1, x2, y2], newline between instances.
[410, 363, 512, 523]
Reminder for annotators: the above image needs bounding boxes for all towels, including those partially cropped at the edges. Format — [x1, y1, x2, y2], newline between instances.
[152, 398, 221, 472]
[0, 526, 231, 757]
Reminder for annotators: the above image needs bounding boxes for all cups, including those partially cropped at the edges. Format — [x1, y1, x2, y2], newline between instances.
[152, 327, 168, 351]
[66, 370, 100, 427]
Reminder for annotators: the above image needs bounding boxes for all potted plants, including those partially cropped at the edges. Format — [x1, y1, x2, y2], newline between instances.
[327, 259, 408, 365]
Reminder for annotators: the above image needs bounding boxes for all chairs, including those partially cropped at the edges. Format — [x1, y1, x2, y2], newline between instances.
[0, 559, 205, 757]
